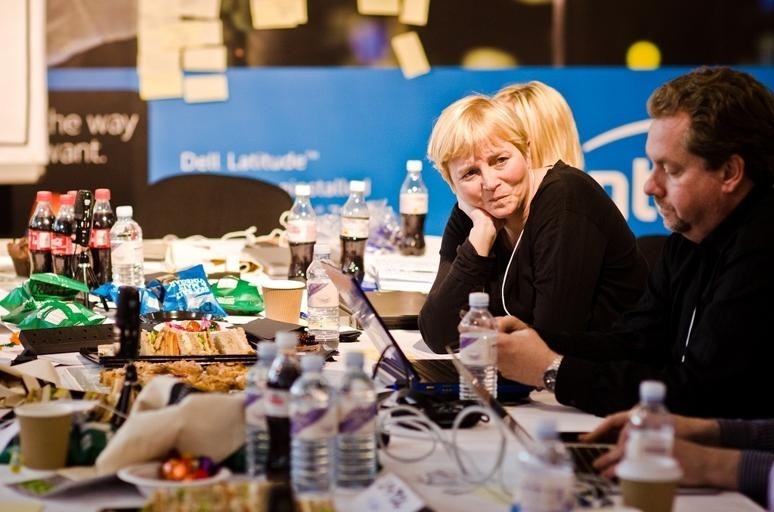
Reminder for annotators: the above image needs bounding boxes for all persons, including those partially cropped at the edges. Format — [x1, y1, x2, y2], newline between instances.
[577, 416, 774, 512]
[492, 82, 585, 173]
[419, 95, 639, 366]
[459, 67, 773, 419]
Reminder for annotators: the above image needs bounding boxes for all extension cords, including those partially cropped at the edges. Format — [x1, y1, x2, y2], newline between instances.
[18, 323, 117, 354]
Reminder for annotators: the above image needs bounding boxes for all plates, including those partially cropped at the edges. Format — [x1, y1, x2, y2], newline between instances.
[377, 381, 480, 429]
[115, 462, 230, 500]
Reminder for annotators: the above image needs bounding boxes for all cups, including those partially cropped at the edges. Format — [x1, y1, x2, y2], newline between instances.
[259, 278, 305, 325]
[14, 402, 74, 470]
[613, 457, 682, 512]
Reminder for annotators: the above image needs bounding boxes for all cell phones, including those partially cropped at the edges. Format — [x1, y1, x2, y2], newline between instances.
[460, 309, 495, 331]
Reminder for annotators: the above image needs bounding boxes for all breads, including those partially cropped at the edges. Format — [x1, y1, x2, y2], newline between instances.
[7, 238, 30, 261]
[149, 481, 332, 512]
[92, 362, 246, 424]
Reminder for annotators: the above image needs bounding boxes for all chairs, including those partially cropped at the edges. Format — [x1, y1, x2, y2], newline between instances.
[637, 230, 667, 264]
[130, 173, 295, 239]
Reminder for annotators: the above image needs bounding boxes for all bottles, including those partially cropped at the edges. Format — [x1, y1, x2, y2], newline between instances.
[512, 418, 576, 511]
[28, 188, 146, 289]
[107, 364, 142, 433]
[249, 331, 375, 496]
[396, 160, 428, 257]
[459, 293, 497, 399]
[625, 378, 676, 474]
[285, 184, 316, 280]
[338, 180, 369, 284]
[304, 243, 338, 353]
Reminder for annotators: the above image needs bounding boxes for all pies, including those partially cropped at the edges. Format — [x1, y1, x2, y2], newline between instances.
[137, 327, 255, 356]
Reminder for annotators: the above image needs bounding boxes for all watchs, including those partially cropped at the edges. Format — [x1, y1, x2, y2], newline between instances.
[541, 355, 562, 393]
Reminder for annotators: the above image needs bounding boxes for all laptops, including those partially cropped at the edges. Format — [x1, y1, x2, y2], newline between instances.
[337, 292, 428, 327]
[321, 260, 531, 399]
[448, 350, 721, 495]
